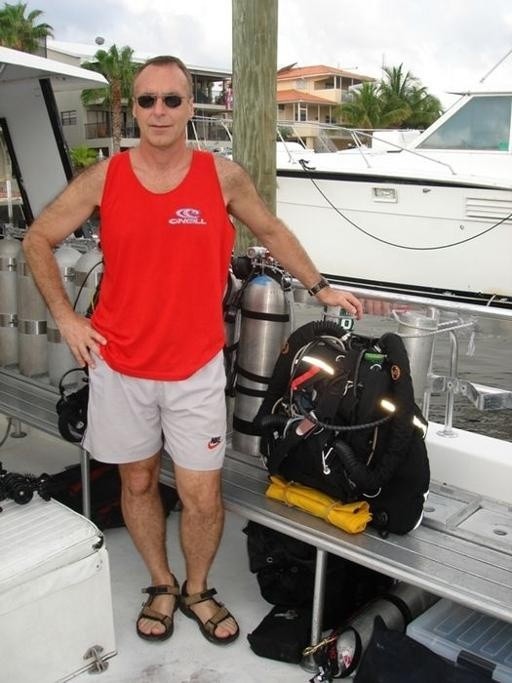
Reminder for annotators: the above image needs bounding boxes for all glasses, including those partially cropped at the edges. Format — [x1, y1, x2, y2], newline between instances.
[132, 92, 188, 109]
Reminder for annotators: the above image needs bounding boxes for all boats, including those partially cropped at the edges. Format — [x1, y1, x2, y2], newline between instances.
[186, 86, 511, 306]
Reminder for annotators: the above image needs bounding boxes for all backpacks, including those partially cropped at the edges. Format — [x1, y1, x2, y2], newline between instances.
[251, 318, 433, 541]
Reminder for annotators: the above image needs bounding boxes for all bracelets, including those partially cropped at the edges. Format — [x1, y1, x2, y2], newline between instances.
[308, 278, 330, 296]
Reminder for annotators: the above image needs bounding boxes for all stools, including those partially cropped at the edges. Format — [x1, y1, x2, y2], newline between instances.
[0, 491, 121, 683]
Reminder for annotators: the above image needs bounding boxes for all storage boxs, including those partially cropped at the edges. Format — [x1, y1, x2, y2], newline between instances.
[404, 596, 510, 682]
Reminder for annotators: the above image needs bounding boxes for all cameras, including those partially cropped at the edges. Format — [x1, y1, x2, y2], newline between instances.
[0, 471, 51, 507]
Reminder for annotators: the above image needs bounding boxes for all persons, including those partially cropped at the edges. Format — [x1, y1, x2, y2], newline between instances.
[21, 57, 363, 643]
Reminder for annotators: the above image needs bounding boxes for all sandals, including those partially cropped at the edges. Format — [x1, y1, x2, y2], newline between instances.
[177, 578, 241, 646]
[135, 572, 181, 643]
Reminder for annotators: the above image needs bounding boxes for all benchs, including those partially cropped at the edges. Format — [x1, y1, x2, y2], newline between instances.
[1, 368, 108, 525]
[143, 422, 511, 681]
